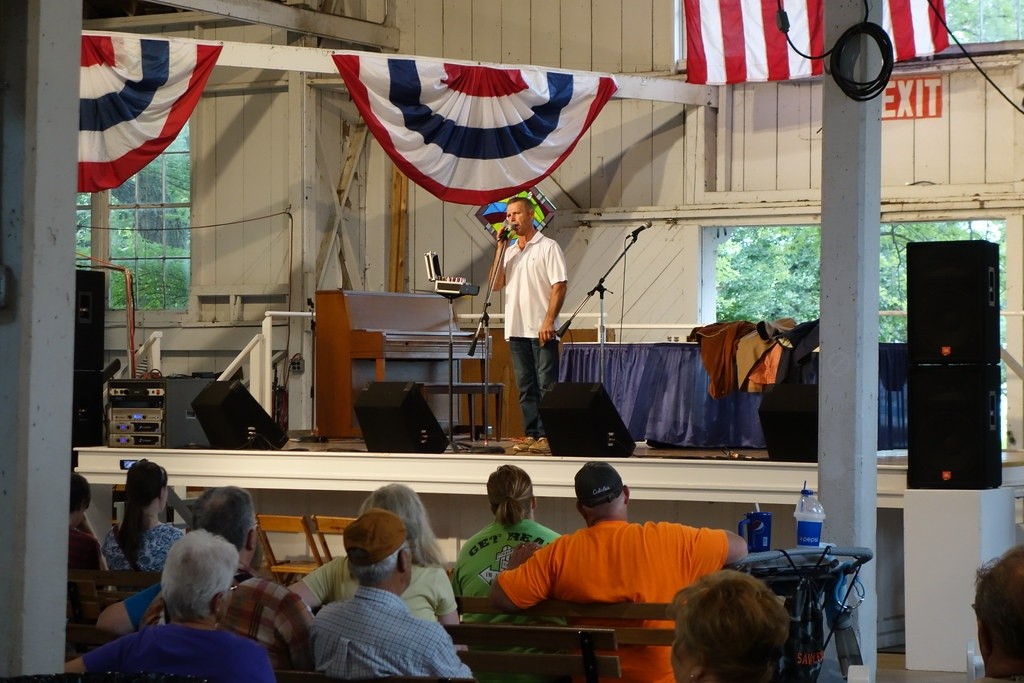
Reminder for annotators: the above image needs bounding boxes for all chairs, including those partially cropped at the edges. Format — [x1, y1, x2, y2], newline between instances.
[249, 514, 360, 590]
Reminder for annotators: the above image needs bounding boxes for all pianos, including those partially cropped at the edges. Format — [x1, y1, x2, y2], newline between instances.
[313, 288, 494, 440]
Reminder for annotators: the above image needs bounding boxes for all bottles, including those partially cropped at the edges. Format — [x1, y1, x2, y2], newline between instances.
[793, 490, 825, 514]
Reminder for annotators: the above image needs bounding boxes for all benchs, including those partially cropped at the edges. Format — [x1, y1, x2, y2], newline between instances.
[0, 595, 680, 683]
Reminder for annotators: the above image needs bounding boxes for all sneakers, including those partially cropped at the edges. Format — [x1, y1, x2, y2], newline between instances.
[512, 437, 535, 451]
[528, 437, 551, 454]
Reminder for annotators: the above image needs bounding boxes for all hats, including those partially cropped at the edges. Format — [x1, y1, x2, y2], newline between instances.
[343, 509, 416, 566]
[575, 462, 622, 507]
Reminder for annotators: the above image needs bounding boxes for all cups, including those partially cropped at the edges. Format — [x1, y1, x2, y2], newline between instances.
[796, 514, 825, 549]
[737, 511, 773, 553]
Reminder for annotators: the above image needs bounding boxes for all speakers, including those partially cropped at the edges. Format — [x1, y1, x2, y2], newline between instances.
[192, 381, 289, 450]
[538, 381, 636, 458]
[906, 364, 1002, 491]
[73, 270, 106, 370]
[354, 381, 449, 454]
[906, 240, 1000, 364]
[71, 370, 104, 448]
[758, 384, 819, 465]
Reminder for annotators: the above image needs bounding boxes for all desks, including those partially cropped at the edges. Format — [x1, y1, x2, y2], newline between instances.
[560, 340, 765, 448]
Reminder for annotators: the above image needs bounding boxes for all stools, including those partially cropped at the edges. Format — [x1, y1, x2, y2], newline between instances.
[411, 383, 507, 442]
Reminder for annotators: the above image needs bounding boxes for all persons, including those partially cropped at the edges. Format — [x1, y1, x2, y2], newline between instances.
[66, 528, 276, 683]
[669, 568, 790, 683]
[451, 465, 568, 683]
[140, 486, 315, 667]
[287, 484, 468, 652]
[310, 509, 472, 679]
[100, 582, 163, 632]
[490, 460, 748, 683]
[101, 459, 184, 573]
[968, 544, 1024, 683]
[68, 473, 101, 569]
[489, 197, 568, 452]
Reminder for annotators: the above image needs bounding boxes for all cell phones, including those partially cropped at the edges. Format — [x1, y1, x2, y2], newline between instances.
[120, 459, 138, 470]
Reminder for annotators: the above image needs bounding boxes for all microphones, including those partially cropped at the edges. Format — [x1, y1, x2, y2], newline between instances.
[498, 224, 515, 241]
[626, 220, 652, 239]
